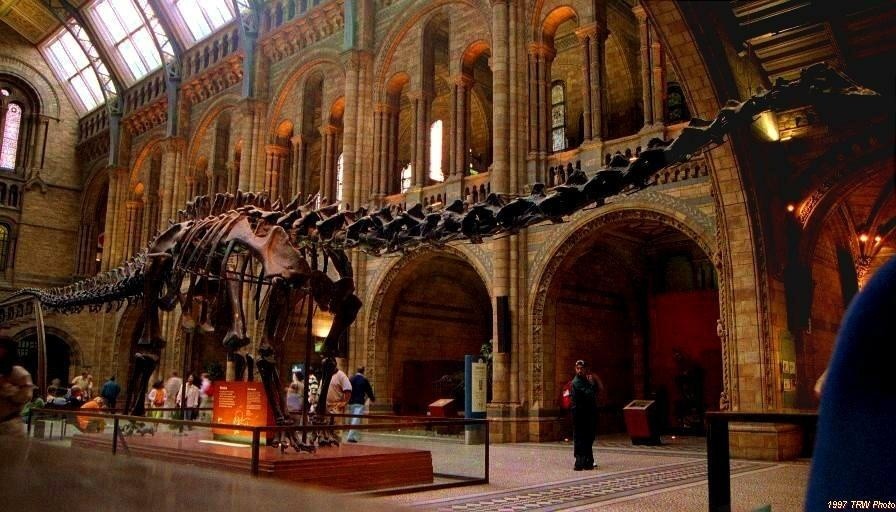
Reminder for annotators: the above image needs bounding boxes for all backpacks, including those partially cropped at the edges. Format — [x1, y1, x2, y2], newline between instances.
[562, 382, 576, 409]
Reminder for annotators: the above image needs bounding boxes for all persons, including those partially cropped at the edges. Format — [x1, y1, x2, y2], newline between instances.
[0, 333, 35, 435]
[343, 364, 375, 444]
[569, 357, 602, 473]
[100, 375, 120, 414]
[285, 370, 305, 426]
[197, 371, 215, 423]
[74, 395, 110, 434]
[162, 370, 182, 419]
[306, 365, 318, 425]
[20, 370, 95, 426]
[175, 372, 199, 433]
[311, 355, 354, 442]
[147, 378, 168, 431]
[562, 373, 575, 444]
[584, 360, 610, 440]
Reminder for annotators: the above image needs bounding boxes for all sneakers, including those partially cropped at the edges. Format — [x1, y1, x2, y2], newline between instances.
[574, 461, 593, 471]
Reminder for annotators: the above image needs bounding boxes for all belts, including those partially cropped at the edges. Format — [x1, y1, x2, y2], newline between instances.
[0, 412, 19, 423]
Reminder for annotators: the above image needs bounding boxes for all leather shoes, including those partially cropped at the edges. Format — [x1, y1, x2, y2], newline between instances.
[348, 440, 358, 443]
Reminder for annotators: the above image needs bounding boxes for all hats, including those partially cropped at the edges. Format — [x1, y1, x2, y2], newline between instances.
[152, 380, 163, 388]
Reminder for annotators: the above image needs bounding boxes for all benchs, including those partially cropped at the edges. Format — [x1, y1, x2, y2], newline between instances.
[32, 415, 73, 440]
